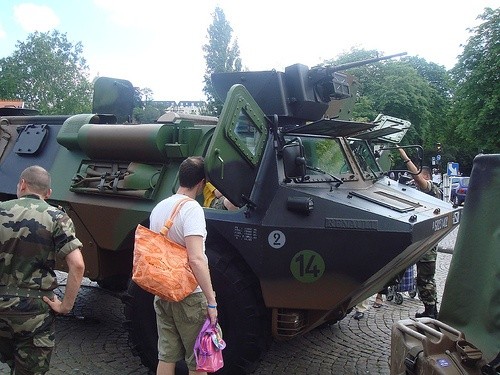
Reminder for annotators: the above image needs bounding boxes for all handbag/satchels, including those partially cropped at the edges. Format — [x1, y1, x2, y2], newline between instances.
[193, 316, 224, 372]
[131, 199, 199, 303]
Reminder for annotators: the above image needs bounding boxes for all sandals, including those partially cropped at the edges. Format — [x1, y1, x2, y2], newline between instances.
[373, 297, 383, 308]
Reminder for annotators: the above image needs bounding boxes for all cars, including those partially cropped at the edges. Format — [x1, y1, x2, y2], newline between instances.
[438, 176, 470, 208]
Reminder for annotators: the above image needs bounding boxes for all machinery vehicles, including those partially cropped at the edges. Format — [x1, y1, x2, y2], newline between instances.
[0, 52, 463, 375]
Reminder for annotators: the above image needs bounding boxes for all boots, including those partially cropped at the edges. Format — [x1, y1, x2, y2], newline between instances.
[415, 302, 438, 319]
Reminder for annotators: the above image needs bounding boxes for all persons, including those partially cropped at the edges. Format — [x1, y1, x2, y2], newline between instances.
[396, 144, 440, 320]
[0, 165, 86, 375]
[372, 293, 382, 308]
[203, 181, 239, 211]
[149, 157, 219, 375]
[354, 298, 369, 320]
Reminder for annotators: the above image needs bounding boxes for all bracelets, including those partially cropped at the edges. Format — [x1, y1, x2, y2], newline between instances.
[208, 304, 217, 309]
